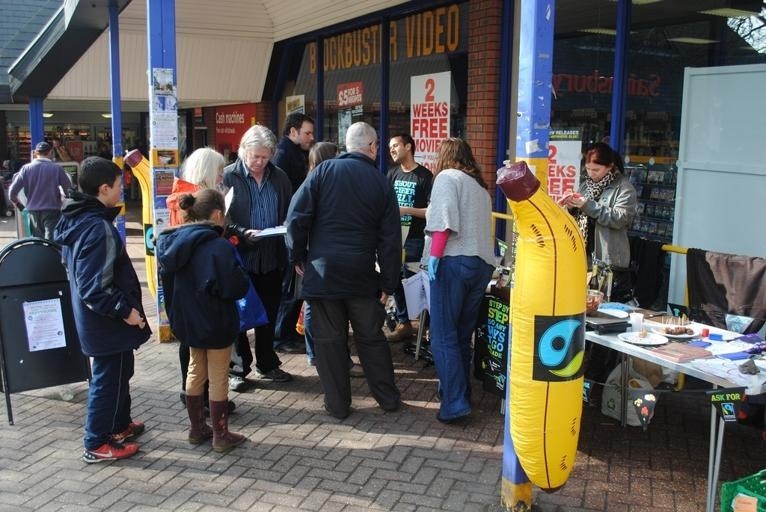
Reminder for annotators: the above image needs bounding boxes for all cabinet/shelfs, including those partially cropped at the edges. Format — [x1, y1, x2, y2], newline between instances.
[5, 126, 92, 166]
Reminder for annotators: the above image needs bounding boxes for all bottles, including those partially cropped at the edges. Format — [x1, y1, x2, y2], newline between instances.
[588, 254, 613, 303]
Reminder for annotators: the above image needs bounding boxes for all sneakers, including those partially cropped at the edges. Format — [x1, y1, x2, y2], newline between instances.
[290, 331, 306, 342]
[82, 440, 139, 464]
[386, 322, 414, 342]
[418, 326, 431, 345]
[114, 421, 144, 444]
[274, 341, 306, 355]
[308, 358, 317, 366]
[350, 364, 366, 377]
[228, 375, 249, 392]
[436, 411, 472, 423]
[256, 367, 292, 382]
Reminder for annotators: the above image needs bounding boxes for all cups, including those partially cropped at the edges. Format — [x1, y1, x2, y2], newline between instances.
[629, 312, 645, 330]
[587, 289, 604, 316]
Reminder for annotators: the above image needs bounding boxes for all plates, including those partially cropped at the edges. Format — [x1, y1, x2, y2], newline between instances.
[254, 231, 287, 238]
[652, 325, 701, 339]
[617, 331, 669, 347]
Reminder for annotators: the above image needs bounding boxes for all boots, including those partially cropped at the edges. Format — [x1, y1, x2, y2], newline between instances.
[184, 394, 212, 445]
[209, 397, 245, 453]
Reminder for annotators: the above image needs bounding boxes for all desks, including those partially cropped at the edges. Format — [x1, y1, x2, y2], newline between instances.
[582, 301, 765, 512]
[402, 256, 513, 419]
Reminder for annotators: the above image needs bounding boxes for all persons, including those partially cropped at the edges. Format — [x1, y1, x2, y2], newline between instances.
[420, 138, 496, 424]
[99, 144, 113, 159]
[51, 139, 70, 162]
[564, 142, 636, 381]
[0, 159, 25, 180]
[8, 142, 72, 244]
[53, 156, 152, 463]
[125, 139, 146, 199]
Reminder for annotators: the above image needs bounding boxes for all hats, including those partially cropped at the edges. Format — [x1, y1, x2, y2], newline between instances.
[35, 142, 50, 152]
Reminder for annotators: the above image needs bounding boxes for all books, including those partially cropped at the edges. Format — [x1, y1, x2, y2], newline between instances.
[652, 342, 713, 363]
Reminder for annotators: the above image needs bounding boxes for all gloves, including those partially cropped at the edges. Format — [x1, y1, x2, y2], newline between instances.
[427, 256, 440, 282]
